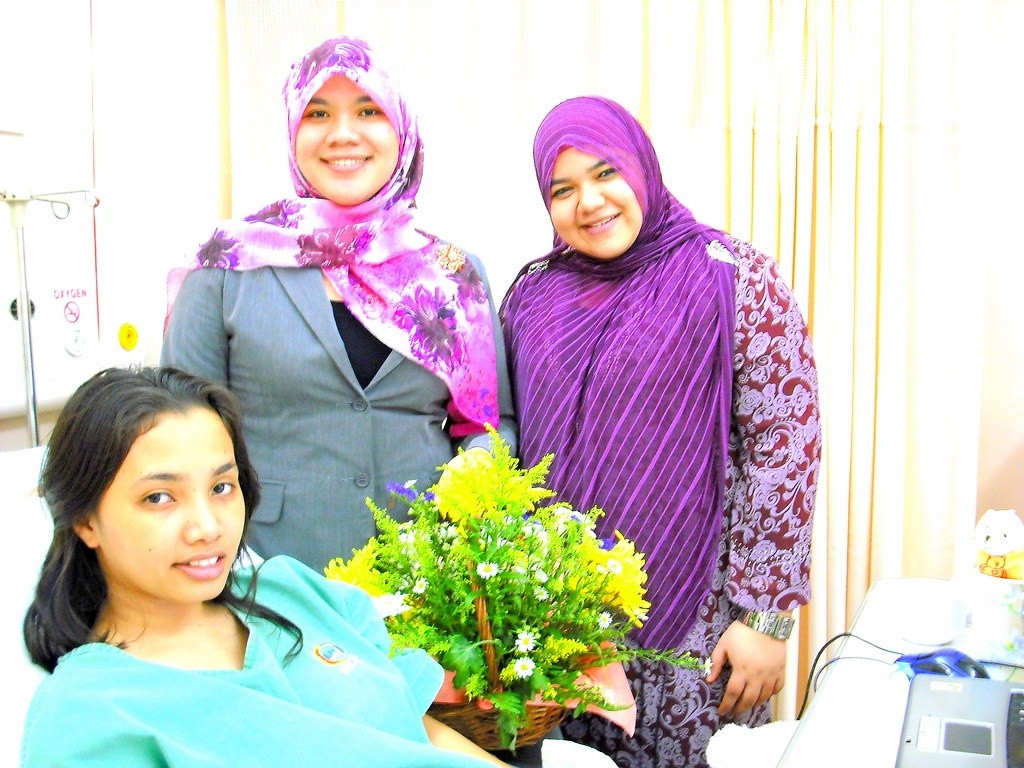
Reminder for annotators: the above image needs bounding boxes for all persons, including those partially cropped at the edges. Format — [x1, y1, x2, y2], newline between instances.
[499, 93, 825, 768]
[160, 35, 515, 583]
[16, 365, 521, 768]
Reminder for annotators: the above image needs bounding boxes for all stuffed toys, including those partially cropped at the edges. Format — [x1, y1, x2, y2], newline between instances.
[969, 507, 1024, 579]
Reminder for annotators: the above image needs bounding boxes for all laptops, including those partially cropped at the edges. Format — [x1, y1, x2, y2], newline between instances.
[895, 672, 1024, 768]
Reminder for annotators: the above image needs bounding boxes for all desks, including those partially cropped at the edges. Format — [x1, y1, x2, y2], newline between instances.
[776, 554, 1024, 768]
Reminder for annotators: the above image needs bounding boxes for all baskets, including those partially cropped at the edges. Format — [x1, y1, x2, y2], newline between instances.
[423, 557, 576, 755]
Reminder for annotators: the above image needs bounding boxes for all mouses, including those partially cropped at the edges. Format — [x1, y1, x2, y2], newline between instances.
[893, 646, 990, 679]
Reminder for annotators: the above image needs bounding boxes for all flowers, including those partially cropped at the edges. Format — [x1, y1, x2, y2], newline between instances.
[324, 422, 714, 756]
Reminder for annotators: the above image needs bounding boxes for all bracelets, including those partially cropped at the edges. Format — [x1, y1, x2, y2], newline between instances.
[739, 604, 795, 641]
